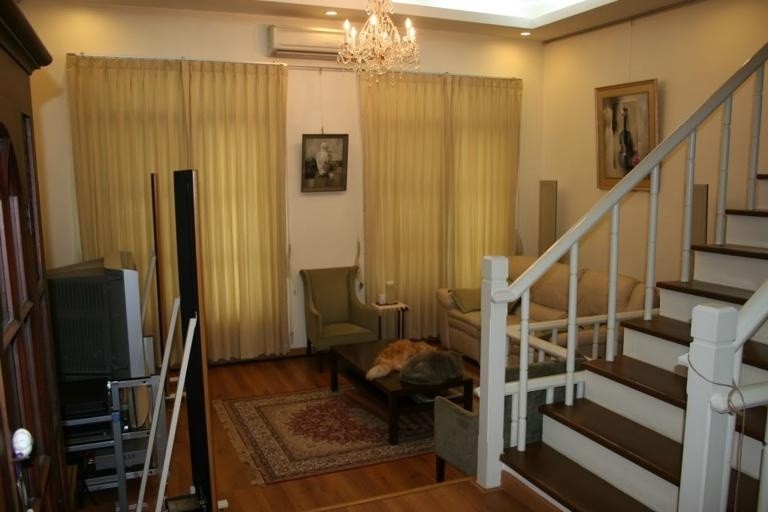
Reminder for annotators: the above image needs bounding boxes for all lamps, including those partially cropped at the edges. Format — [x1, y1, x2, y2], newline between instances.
[335, 0, 421, 88]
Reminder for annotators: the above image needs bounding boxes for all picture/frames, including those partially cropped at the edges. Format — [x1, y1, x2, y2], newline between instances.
[593, 76, 661, 195]
[298, 131, 349, 194]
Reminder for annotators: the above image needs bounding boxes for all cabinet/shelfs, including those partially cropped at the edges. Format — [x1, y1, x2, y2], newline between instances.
[59, 334, 172, 497]
[2, 0, 82, 512]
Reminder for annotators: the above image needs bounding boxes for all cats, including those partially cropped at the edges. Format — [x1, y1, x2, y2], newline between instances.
[366, 338, 438, 381]
[398, 346, 465, 386]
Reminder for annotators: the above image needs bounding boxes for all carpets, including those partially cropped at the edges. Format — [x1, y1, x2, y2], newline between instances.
[210, 370, 480, 488]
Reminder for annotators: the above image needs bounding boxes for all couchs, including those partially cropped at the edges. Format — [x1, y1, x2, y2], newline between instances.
[432, 253, 656, 379]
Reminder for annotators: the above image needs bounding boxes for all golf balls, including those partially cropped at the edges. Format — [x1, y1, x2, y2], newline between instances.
[13, 428, 34, 455]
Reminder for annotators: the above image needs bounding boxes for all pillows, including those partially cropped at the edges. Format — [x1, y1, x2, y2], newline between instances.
[508, 278, 521, 315]
[447, 287, 481, 317]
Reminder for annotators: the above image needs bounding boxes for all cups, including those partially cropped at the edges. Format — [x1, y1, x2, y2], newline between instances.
[378, 293, 386, 305]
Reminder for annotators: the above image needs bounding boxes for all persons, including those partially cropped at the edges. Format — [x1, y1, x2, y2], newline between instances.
[316, 142, 331, 176]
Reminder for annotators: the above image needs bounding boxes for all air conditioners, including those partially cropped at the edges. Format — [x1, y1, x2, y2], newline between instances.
[266, 23, 388, 66]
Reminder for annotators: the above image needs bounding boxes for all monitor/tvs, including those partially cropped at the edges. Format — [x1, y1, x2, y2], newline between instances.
[45, 250, 146, 380]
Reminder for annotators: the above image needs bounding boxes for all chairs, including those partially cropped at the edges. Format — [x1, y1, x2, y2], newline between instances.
[431, 357, 589, 484]
[297, 264, 381, 377]
